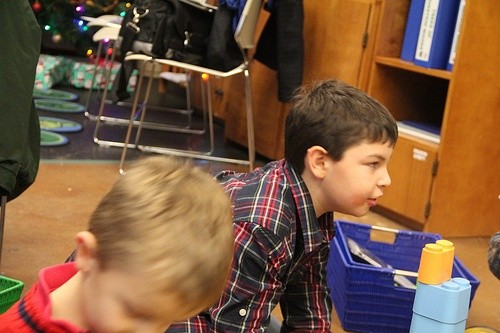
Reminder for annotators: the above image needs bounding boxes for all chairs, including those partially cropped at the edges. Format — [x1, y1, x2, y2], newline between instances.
[81, 0, 270, 180]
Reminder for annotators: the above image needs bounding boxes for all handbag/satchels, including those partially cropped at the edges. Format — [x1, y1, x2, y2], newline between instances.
[152, 0, 243, 72]
[112, 0, 171, 105]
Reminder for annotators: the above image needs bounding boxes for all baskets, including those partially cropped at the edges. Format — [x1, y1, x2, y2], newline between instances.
[325, 219, 480, 333]
[0, 276, 24, 314]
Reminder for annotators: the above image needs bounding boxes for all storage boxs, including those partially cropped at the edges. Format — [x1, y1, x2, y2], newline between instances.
[325, 220, 481, 333]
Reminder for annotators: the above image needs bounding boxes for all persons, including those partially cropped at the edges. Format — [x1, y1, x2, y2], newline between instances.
[0, 0, 43, 202]
[65, 79, 399, 333]
[0, 157, 234, 333]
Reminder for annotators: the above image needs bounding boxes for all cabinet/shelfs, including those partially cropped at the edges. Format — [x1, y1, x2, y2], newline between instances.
[190, 0, 500, 239]
